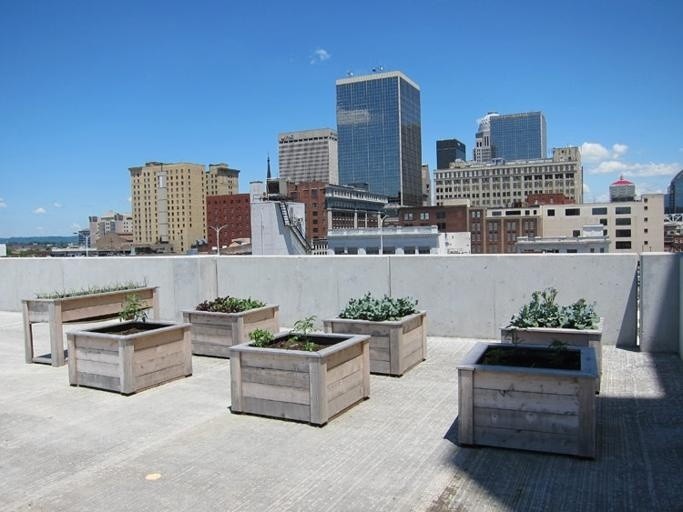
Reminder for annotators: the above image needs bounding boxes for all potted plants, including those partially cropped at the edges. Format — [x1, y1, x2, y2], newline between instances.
[64, 292, 194, 397]
[456, 328, 602, 460]
[21, 277, 160, 368]
[180, 295, 279, 360]
[500, 286, 606, 396]
[321, 290, 427, 378]
[227, 314, 370, 429]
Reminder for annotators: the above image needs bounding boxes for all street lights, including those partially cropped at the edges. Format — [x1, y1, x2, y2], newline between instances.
[204, 224, 230, 254]
[73, 230, 100, 255]
[373, 213, 390, 254]
